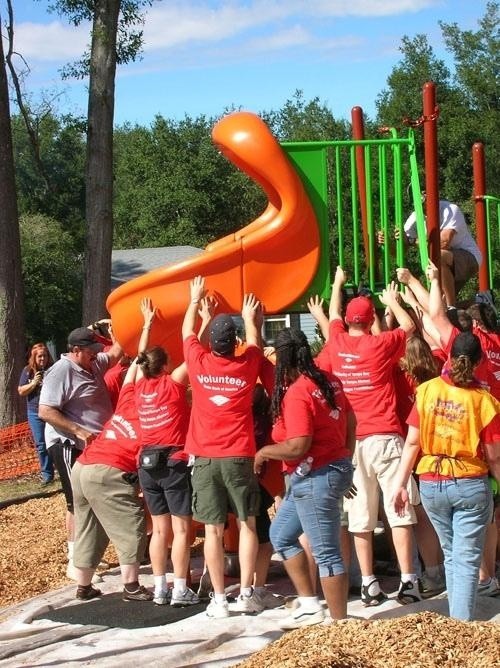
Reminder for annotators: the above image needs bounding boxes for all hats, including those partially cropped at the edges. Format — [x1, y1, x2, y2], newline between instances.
[67, 327, 105, 350]
[208, 313, 235, 354]
[346, 296, 375, 324]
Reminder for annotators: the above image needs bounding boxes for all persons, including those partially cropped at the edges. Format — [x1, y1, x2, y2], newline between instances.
[93, 344, 153, 566]
[254, 265, 500, 620]
[181, 274, 264, 620]
[38, 326, 124, 584]
[18, 343, 54, 489]
[378, 182, 483, 312]
[197, 383, 285, 608]
[134, 297, 219, 608]
[71, 353, 154, 602]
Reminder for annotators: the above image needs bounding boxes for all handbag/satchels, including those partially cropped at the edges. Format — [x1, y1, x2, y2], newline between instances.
[138, 443, 183, 471]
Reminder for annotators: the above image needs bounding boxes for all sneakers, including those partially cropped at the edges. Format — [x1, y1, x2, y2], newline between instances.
[395, 579, 423, 606]
[121, 585, 155, 602]
[263, 590, 286, 609]
[169, 587, 201, 607]
[152, 588, 172, 605]
[66, 562, 104, 584]
[97, 561, 109, 570]
[205, 591, 230, 619]
[235, 588, 264, 614]
[360, 577, 389, 607]
[420, 574, 446, 598]
[75, 585, 102, 601]
[278, 603, 325, 630]
[476, 577, 500, 597]
